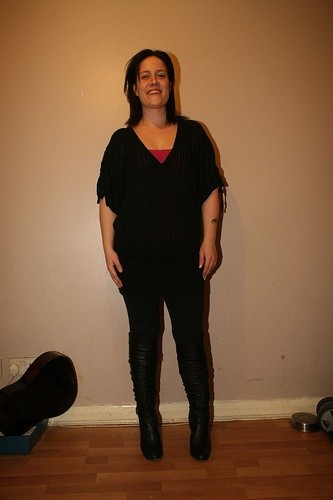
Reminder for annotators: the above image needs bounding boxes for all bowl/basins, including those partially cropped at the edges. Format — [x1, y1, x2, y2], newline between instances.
[289, 412, 321, 433]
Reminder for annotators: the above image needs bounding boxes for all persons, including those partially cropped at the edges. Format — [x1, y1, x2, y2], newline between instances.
[95, 48, 223, 461]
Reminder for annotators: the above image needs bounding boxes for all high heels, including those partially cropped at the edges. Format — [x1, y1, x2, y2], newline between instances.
[188, 407, 212, 460]
[137, 412, 163, 459]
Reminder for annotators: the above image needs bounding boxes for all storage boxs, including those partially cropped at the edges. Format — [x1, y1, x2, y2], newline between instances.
[0, 417, 48, 456]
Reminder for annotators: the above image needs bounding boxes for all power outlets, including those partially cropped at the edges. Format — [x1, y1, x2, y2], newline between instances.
[9, 357, 36, 376]
